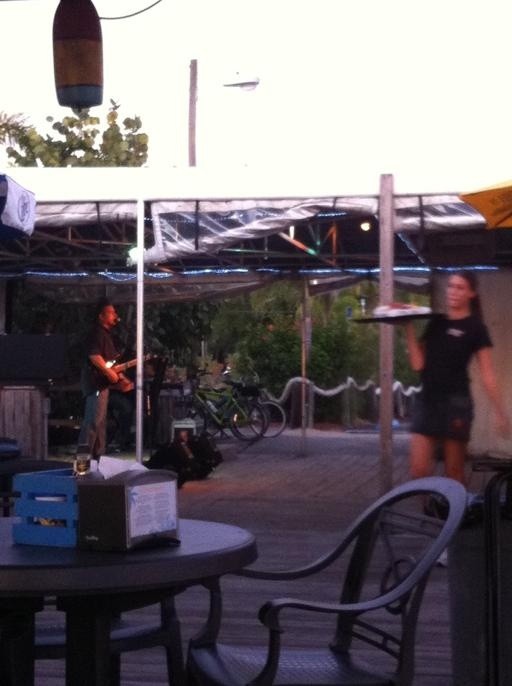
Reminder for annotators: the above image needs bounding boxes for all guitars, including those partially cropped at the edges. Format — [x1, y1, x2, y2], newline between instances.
[85, 348, 160, 391]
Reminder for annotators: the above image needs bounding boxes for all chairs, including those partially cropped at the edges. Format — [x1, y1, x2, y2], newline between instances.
[0, 446, 184, 685]
[181, 475, 467, 686]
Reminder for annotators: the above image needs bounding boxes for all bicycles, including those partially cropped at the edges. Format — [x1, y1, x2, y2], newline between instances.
[153, 362, 288, 444]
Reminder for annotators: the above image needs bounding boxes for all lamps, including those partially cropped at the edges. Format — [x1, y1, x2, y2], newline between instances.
[48, 0, 107, 120]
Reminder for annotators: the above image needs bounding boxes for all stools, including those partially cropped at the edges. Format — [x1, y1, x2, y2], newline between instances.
[166, 417, 205, 445]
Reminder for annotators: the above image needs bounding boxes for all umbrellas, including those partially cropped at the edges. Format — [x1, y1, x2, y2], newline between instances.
[457, 182, 512, 233]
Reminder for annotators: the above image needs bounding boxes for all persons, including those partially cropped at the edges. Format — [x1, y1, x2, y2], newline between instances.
[402, 269, 511, 567]
[75, 296, 134, 460]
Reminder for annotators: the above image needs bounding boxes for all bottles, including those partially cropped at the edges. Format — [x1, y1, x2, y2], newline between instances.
[74, 443, 92, 477]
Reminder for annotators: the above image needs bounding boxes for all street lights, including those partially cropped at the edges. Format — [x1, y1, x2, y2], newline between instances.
[188, 73, 260, 166]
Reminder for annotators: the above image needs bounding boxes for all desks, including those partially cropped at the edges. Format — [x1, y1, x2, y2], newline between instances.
[0, 509, 257, 686]
